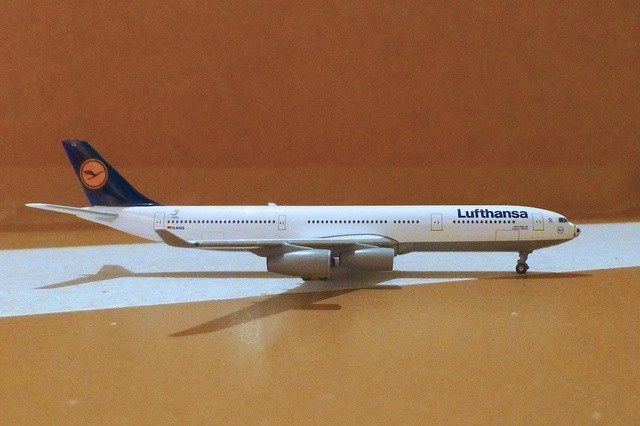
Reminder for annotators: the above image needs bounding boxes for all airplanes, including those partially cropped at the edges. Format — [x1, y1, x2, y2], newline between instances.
[25, 140, 581, 285]
